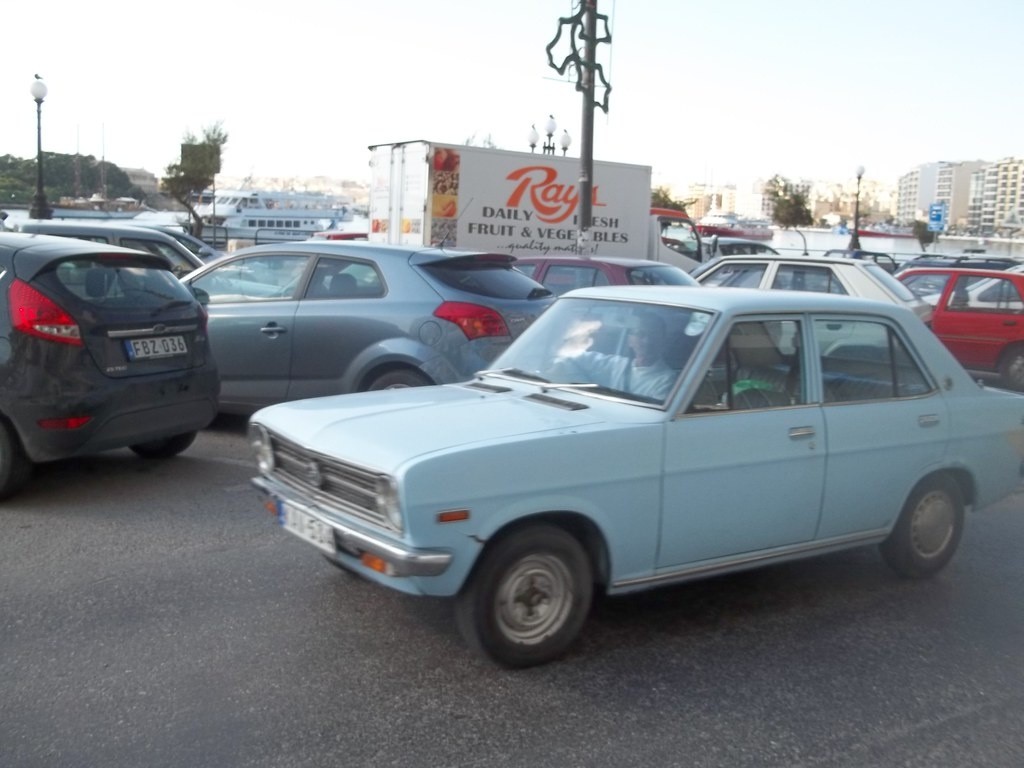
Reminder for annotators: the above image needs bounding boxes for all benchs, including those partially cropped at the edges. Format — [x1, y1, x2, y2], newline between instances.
[735, 363, 907, 408]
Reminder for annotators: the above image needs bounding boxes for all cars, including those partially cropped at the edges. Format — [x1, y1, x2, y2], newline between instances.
[0, 218, 224, 499]
[244, 283, 1024, 674]
[686, 253, 935, 327]
[510, 255, 705, 296]
[310, 231, 368, 242]
[177, 241, 557, 418]
[890, 267, 1024, 390]
[703, 239, 1024, 272]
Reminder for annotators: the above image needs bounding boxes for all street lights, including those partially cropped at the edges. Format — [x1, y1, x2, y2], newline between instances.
[28, 73, 53, 219]
[853, 165, 869, 248]
[527, 114, 573, 156]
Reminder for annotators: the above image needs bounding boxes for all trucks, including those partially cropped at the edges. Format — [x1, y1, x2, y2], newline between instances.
[368, 139, 718, 279]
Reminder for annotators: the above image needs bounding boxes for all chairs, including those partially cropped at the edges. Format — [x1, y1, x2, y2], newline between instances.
[950, 288, 970, 306]
[328, 273, 357, 297]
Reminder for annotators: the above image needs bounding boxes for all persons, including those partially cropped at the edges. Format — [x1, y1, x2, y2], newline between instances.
[541, 314, 679, 400]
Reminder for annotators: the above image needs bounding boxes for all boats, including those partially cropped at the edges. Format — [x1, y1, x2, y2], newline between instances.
[693, 203, 778, 239]
[858, 218, 918, 239]
[199, 188, 344, 243]
